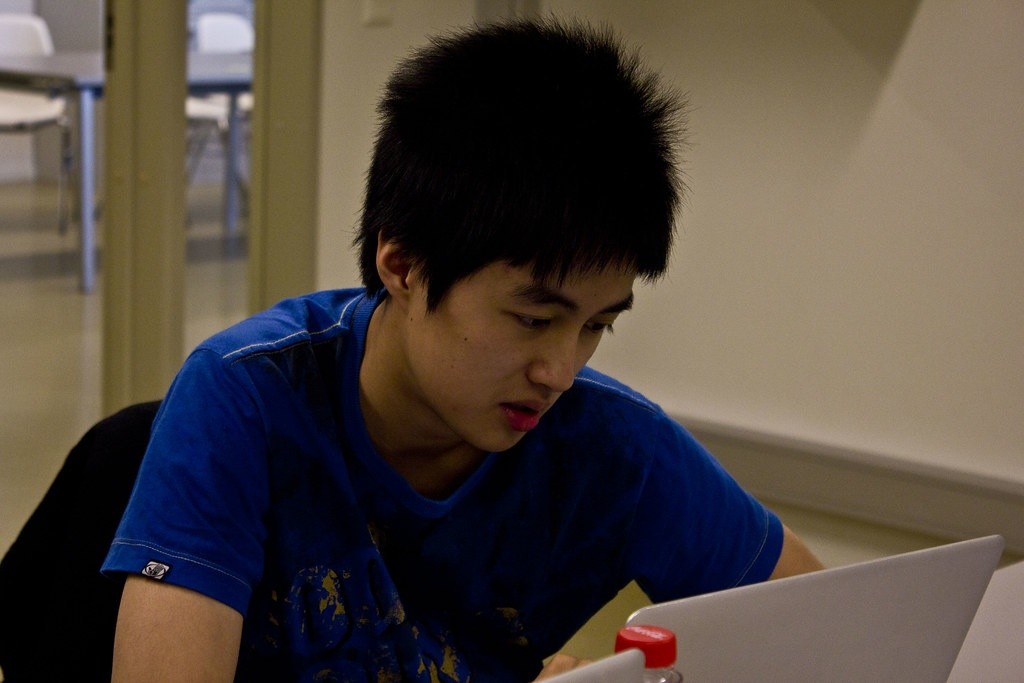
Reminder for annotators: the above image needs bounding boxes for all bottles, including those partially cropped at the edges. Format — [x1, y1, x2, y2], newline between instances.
[614, 624, 684, 683]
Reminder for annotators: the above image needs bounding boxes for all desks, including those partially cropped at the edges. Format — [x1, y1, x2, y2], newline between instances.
[0, 50, 252, 293]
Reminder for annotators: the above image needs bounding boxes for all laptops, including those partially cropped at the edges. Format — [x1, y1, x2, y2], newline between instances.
[623, 533, 1004, 683]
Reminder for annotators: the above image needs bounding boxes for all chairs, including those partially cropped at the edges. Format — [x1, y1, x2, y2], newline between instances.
[0, 402, 160, 683]
[0, 14, 71, 238]
[187, 12, 255, 190]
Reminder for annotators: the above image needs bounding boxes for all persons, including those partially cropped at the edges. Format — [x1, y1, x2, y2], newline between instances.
[99, 19, 826, 682]
